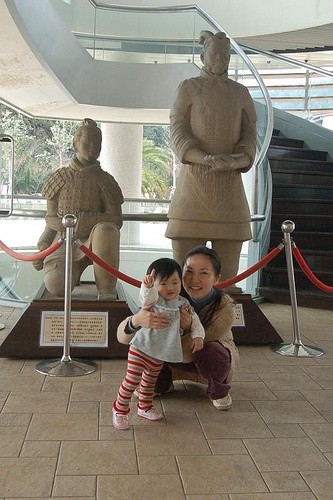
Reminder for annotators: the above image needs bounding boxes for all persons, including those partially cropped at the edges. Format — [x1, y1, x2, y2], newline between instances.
[163, 30, 258, 284]
[31, 117, 124, 301]
[116, 245, 241, 411]
[112, 258, 206, 430]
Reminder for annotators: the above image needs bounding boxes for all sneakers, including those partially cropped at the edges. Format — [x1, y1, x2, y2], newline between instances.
[112, 407, 130, 429]
[212, 394, 233, 411]
[135, 407, 163, 420]
[133, 380, 173, 397]
